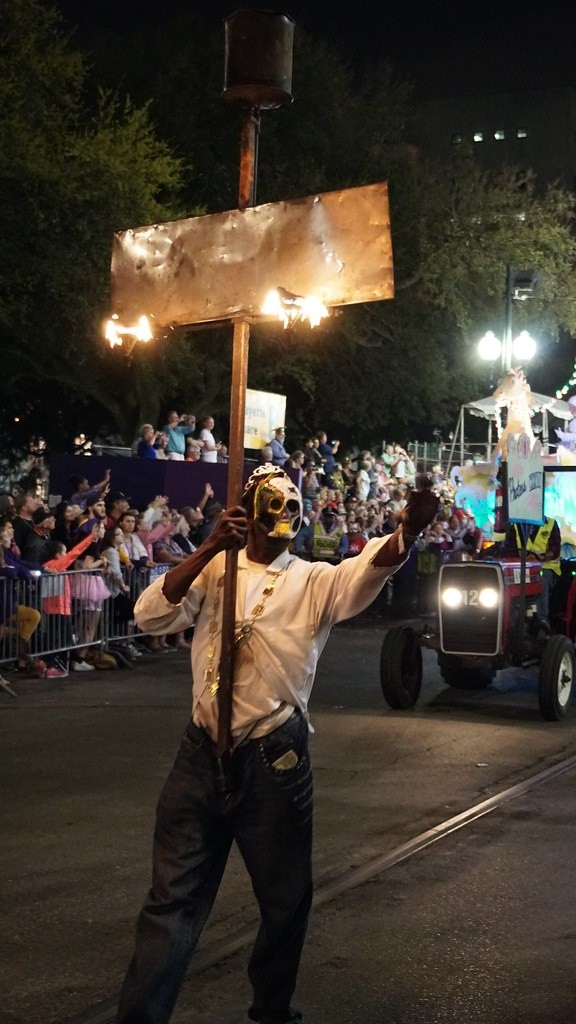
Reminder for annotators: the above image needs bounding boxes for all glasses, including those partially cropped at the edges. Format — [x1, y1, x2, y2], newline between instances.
[114, 533, 125, 536]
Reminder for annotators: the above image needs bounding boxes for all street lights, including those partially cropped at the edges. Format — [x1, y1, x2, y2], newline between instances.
[473, 266, 539, 443]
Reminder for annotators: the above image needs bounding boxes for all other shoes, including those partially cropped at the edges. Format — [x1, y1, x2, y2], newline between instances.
[10, 639, 193, 678]
[539, 620, 551, 634]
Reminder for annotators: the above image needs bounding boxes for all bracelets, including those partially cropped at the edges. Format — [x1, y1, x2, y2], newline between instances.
[396, 521, 422, 556]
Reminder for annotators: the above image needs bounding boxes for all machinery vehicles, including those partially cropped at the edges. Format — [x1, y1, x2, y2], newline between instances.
[379, 461, 576, 723]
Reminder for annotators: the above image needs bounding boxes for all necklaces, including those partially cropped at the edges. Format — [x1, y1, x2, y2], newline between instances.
[203, 560, 288, 696]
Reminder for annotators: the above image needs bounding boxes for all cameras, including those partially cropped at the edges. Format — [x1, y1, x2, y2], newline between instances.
[184, 415, 192, 420]
[331, 440, 336, 444]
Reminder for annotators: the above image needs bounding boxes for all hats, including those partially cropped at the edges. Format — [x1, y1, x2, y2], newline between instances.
[106, 491, 132, 506]
[205, 501, 228, 520]
[347, 497, 358, 504]
[33, 506, 53, 525]
[73, 514, 98, 537]
[276, 427, 284, 434]
[82, 494, 106, 515]
[322, 506, 339, 518]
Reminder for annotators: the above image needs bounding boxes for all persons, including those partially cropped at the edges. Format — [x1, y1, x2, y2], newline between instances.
[114, 462, 442, 1024]
[506, 496, 563, 634]
[3, 407, 482, 677]
